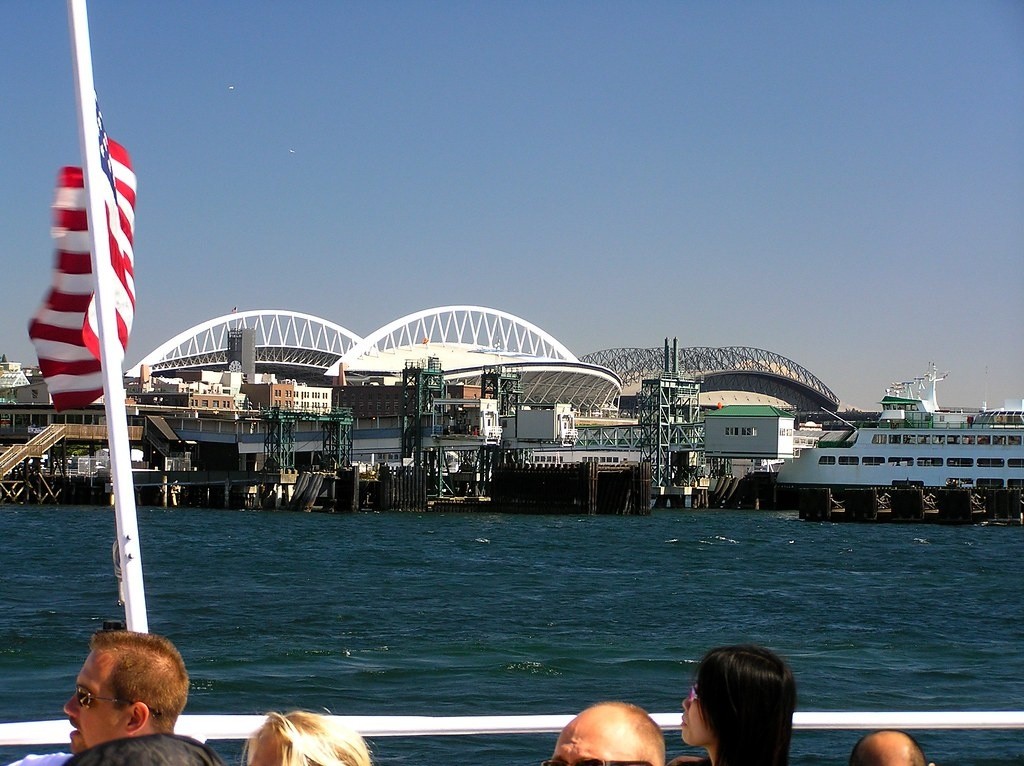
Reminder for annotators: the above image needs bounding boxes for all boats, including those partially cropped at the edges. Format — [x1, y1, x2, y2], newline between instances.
[775, 362, 1024, 489]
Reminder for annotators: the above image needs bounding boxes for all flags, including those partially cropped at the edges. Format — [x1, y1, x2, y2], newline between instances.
[24, 89, 135, 415]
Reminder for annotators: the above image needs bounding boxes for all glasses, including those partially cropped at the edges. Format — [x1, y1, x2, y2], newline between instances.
[72, 682, 163, 718]
[685, 687, 702, 702]
[538, 760, 651, 766]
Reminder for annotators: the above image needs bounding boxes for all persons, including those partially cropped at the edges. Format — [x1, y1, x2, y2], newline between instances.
[246, 710, 370, 766]
[63, 632, 189, 754]
[680, 645, 795, 766]
[847, 730, 938, 766]
[542, 701, 666, 766]
